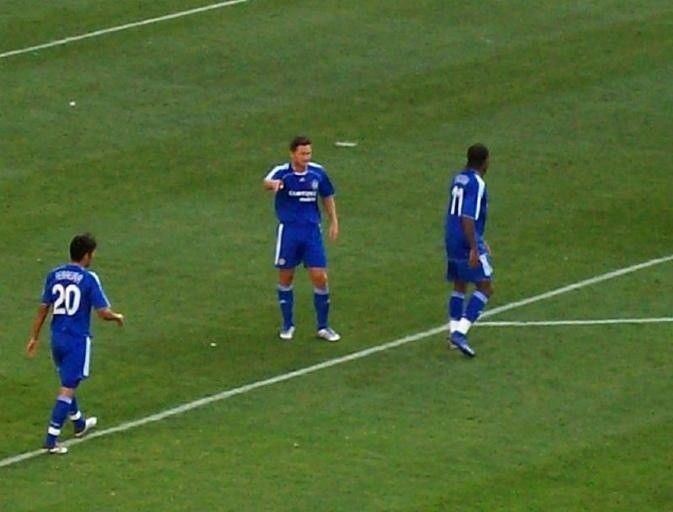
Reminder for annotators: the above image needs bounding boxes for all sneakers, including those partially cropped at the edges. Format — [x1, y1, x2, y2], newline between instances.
[41, 444, 68, 454]
[317, 327, 341, 341]
[74, 416, 97, 438]
[280, 324, 296, 339]
[450, 331, 475, 356]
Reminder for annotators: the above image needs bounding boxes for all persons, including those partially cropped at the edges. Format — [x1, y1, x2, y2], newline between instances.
[263, 136, 341, 342]
[443, 142, 495, 357]
[24, 230, 127, 455]
